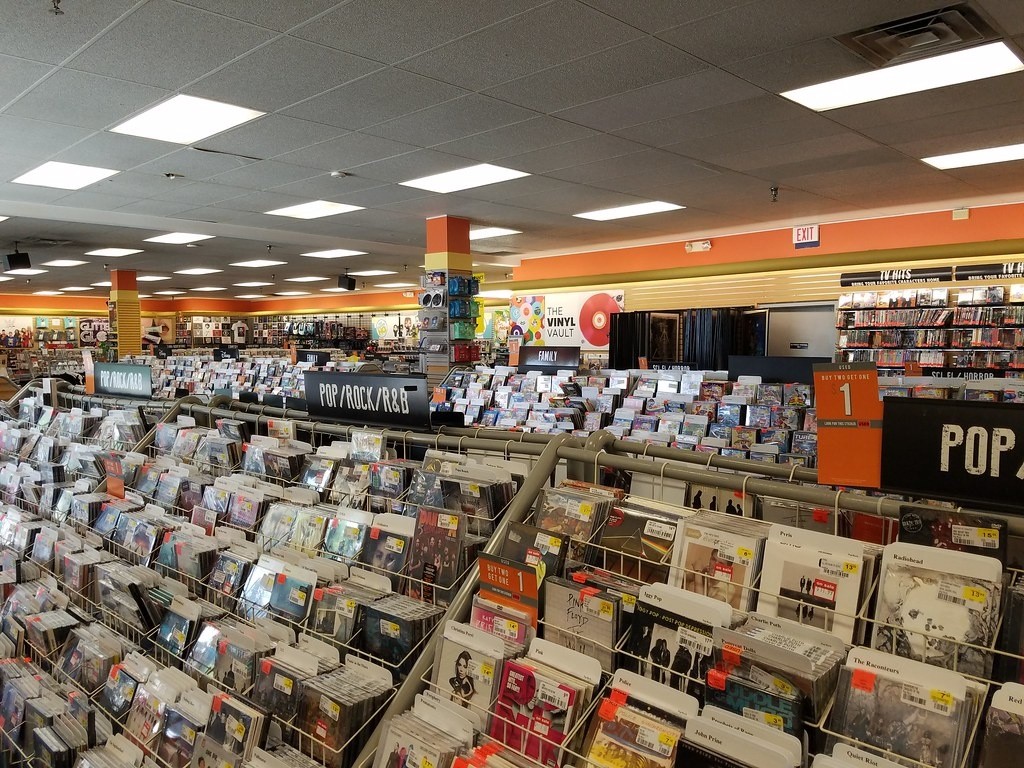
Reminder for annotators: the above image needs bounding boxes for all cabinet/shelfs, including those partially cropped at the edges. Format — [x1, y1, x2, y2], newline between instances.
[0, 277, 1024, 768]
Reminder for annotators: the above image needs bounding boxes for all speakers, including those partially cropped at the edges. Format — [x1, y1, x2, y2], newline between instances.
[4, 253, 31, 271]
[338, 276, 356, 291]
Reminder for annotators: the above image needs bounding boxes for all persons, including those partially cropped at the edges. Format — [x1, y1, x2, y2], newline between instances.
[384, 742, 413, 768]
[197, 757, 205, 768]
[0, 328, 33, 348]
[650, 639, 707, 697]
[449, 651, 474, 708]
[795, 576, 814, 621]
[214, 664, 247, 695]
[511, 675, 552, 728]
[685, 549, 736, 603]
[852, 708, 934, 768]
[208, 712, 248, 753]
[379, 467, 399, 493]
[693, 491, 743, 516]
[370, 540, 397, 578]
[204, 324, 210, 336]
[159, 325, 169, 340]
[128, 705, 157, 743]
[215, 323, 219, 329]
[409, 536, 455, 591]
[605, 715, 639, 768]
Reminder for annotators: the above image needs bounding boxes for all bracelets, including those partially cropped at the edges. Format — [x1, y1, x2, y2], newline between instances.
[695, 569, 702, 572]
